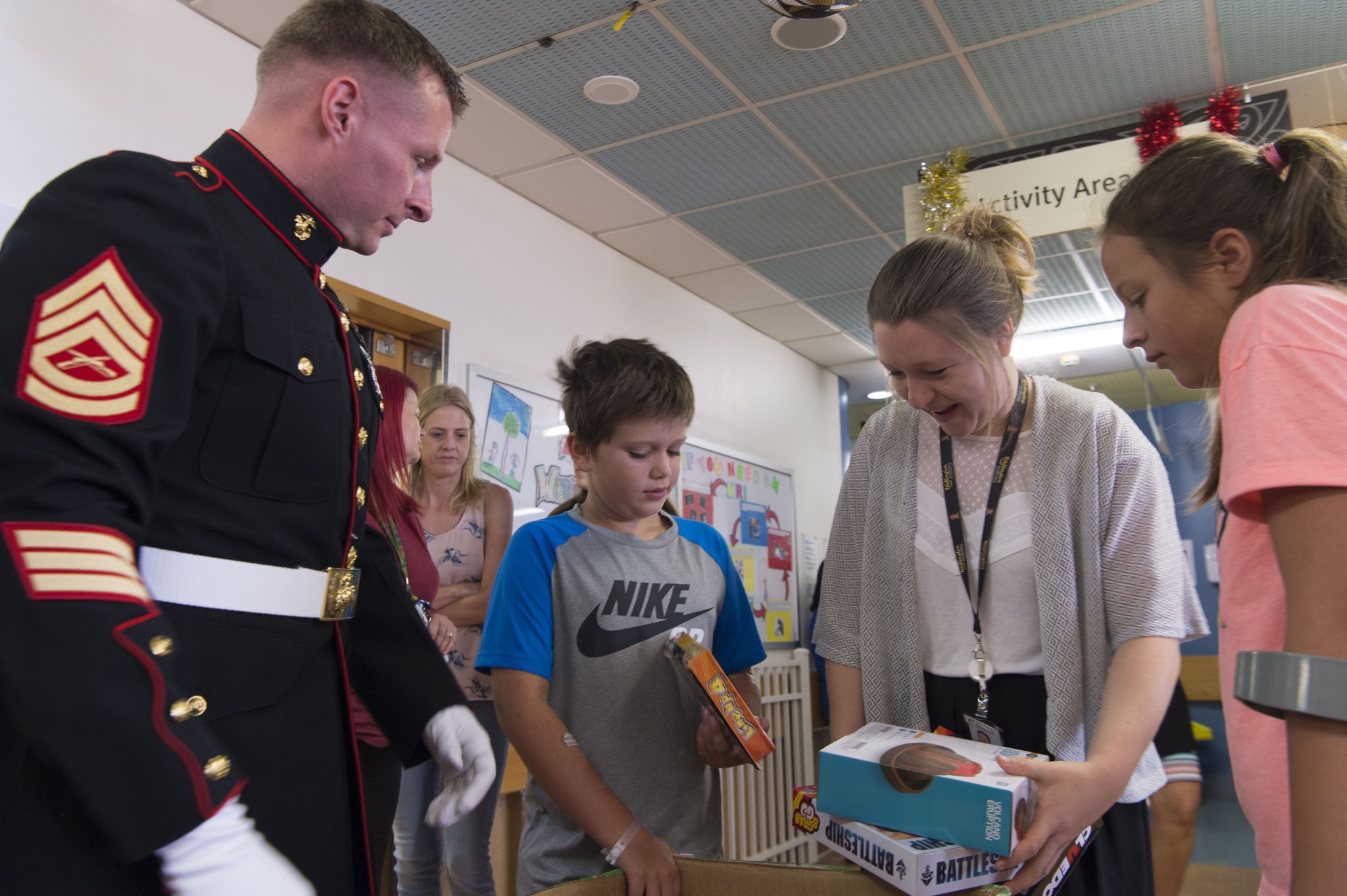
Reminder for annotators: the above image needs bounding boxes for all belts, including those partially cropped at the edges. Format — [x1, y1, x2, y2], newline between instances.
[138, 544, 361, 622]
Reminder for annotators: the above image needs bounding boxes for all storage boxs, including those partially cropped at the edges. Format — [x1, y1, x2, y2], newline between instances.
[664, 633, 778, 770]
[791, 785, 1024, 896]
[1016, 819, 1105, 896]
[816, 722, 1048, 857]
[529, 855, 1011, 896]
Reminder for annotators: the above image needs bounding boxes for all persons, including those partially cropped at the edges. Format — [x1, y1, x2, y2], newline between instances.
[474, 339, 771, 896]
[977, 729, 990, 744]
[1101, 125, 1347, 896]
[345, 367, 456, 896]
[1, 1, 500, 895]
[809, 209, 1210, 896]
[390, 387, 513, 896]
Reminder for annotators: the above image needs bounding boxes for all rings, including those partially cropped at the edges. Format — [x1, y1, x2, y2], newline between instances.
[447, 632, 453, 639]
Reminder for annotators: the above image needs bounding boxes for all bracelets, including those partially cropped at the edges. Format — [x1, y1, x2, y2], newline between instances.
[601, 819, 641, 866]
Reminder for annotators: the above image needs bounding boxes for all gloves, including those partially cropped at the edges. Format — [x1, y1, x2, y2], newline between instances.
[424, 707, 496, 828]
[154, 793, 316, 896]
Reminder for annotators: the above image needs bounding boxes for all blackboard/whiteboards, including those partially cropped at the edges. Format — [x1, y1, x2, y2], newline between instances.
[463, 360, 805, 651]
